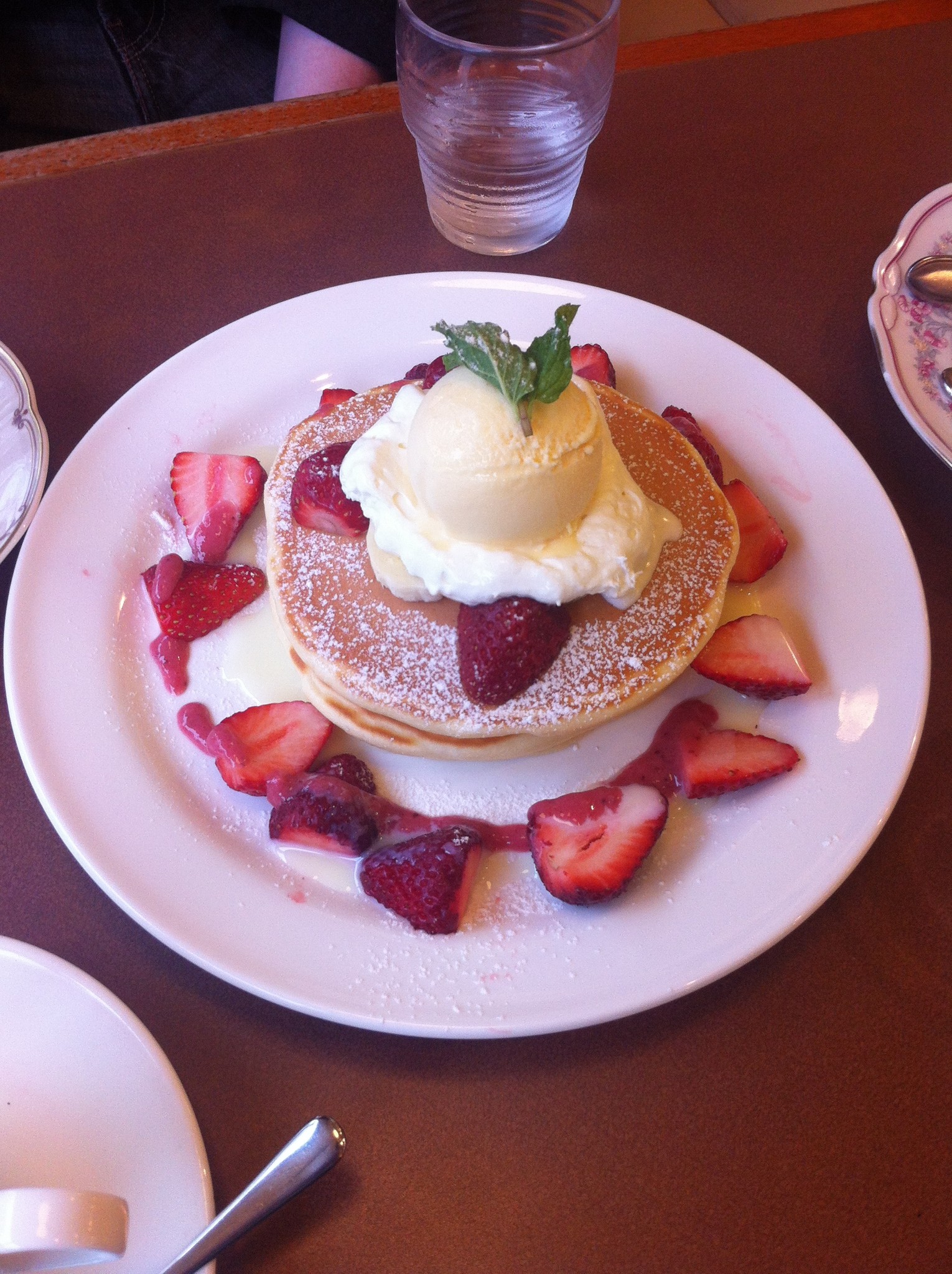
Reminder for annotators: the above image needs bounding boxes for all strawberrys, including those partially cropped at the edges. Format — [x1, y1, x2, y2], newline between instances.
[144, 341, 814, 939]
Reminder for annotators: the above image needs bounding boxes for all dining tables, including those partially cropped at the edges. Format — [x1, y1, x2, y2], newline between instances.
[0, 0, 952, 1274]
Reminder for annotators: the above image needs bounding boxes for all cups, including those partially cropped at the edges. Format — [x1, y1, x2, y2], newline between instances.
[398, 0, 620, 257]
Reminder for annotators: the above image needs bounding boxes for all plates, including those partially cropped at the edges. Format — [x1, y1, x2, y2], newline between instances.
[1, 936, 217, 1273]
[865, 183, 952, 469]
[4, 271, 930, 1039]
[1, 343, 47, 567]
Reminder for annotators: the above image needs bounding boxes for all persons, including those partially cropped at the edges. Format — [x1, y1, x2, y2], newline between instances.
[0, 0, 397, 151]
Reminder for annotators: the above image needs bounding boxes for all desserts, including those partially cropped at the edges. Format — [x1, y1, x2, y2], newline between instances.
[259, 300, 737, 758]
